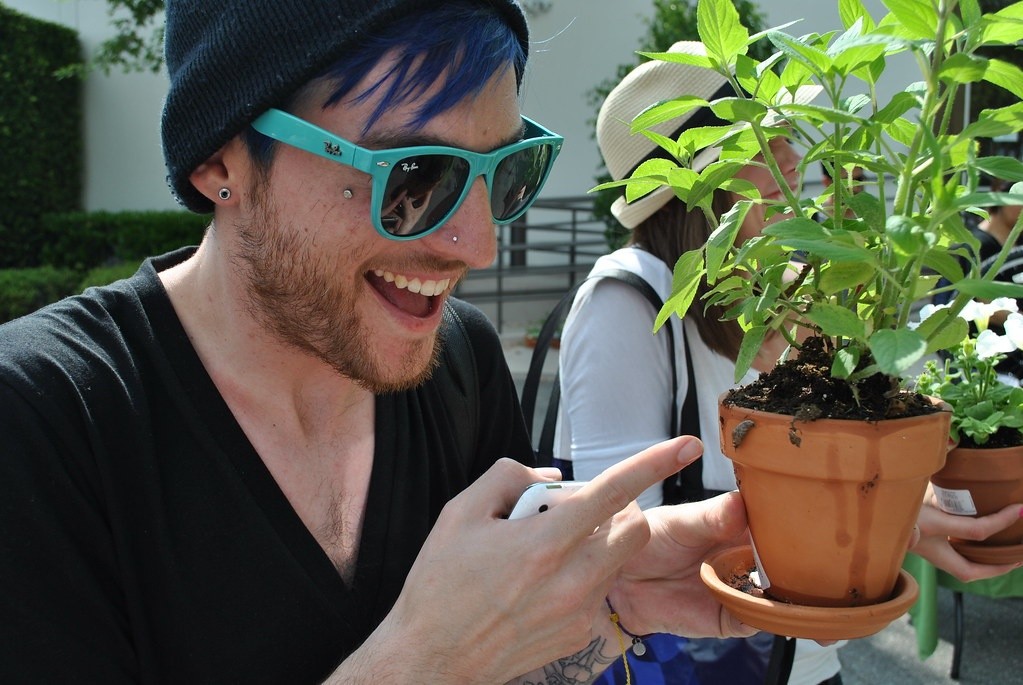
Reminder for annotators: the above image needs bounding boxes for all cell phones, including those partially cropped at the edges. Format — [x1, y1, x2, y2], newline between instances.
[508, 481, 590, 520]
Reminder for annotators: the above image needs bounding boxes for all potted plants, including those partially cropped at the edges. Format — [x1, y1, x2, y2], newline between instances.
[588, 0, 1023, 639]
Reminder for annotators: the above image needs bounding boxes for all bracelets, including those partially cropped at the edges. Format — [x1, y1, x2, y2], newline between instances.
[604, 597, 653, 685]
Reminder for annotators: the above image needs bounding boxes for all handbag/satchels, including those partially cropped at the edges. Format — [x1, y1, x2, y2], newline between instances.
[519, 270, 797, 685]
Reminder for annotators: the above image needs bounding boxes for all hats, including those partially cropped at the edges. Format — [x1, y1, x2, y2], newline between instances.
[161, 0, 529, 214]
[594, 40, 823, 230]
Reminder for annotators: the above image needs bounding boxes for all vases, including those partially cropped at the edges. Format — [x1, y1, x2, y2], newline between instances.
[930, 446, 1023, 564]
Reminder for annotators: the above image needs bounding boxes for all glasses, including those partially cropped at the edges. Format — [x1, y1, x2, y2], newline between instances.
[248, 109, 564, 241]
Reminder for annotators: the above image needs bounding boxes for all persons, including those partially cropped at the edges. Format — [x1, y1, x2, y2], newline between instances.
[553, 39, 1023, 685]
[926, 177, 1023, 385]
[802, 157, 867, 224]
[0, 0, 839, 685]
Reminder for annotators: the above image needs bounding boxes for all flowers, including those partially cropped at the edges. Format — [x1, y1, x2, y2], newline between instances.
[902, 297, 1023, 445]
